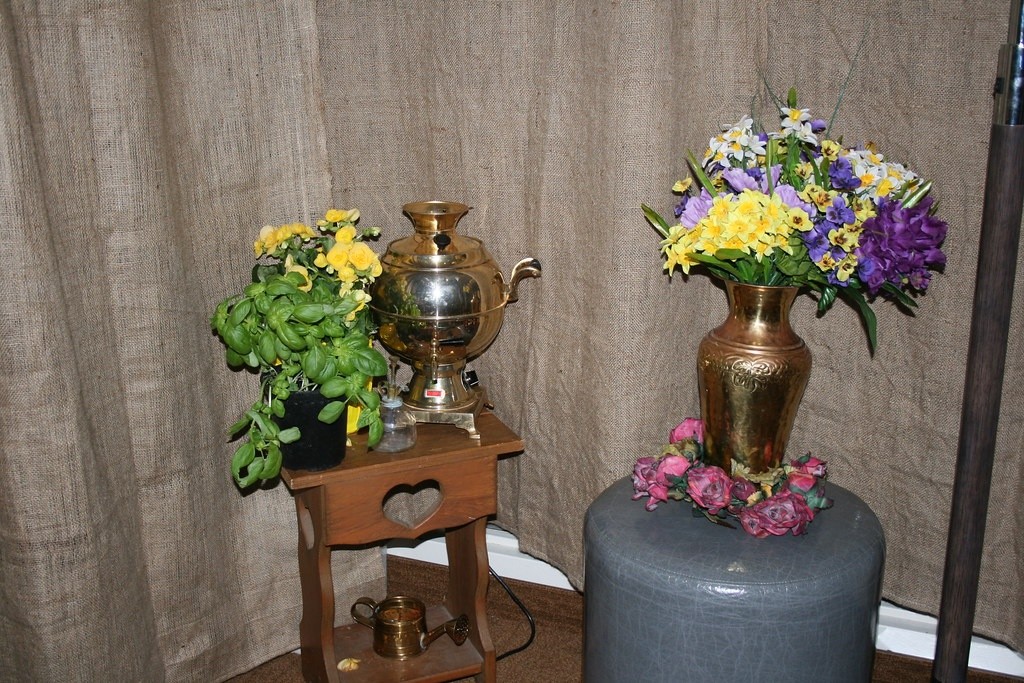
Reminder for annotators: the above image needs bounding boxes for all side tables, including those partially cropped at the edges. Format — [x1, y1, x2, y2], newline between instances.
[276, 406, 524, 683]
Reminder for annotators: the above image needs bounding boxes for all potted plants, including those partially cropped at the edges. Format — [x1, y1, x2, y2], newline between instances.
[206, 271, 387, 490]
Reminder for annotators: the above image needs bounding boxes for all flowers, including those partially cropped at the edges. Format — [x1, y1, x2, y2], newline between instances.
[629, 417, 833, 539]
[639, 80, 950, 356]
[248, 208, 384, 327]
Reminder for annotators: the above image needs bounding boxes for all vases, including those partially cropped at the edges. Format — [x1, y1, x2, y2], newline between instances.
[694, 279, 812, 503]
